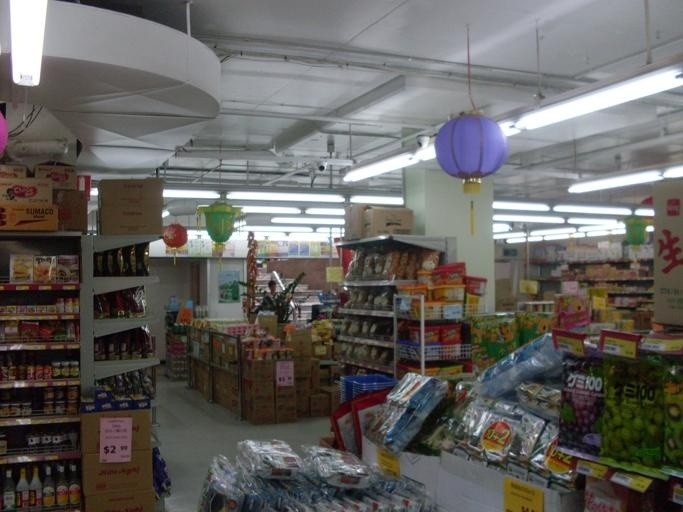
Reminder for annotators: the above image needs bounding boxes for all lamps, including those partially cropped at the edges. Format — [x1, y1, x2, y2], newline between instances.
[98, 189, 404, 215]
[342, 64, 682, 183]
[493, 200, 656, 217]
[569, 166, 683, 195]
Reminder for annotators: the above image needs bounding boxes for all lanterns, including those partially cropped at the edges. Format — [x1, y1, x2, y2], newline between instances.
[163, 224, 188, 265]
[623, 214, 648, 259]
[195, 202, 245, 266]
[433, 112, 509, 238]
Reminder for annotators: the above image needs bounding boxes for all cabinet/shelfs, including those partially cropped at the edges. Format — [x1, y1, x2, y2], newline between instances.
[526, 256, 653, 336]
[338, 234, 458, 392]
[0, 232, 169, 511]
[392, 292, 490, 387]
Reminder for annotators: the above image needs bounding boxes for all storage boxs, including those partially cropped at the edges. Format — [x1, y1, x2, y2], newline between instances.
[652, 175, 682, 328]
[344, 205, 371, 242]
[186, 322, 341, 426]
[361, 205, 413, 236]
[435, 446, 581, 511]
[76, 408, 158, 512]
[0, 163, 167, 232]
[363, 432, 440, 511]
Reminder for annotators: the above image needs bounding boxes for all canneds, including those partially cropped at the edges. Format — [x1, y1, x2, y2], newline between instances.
[0, 434, 8, 455]
[0, 360, 79, 417]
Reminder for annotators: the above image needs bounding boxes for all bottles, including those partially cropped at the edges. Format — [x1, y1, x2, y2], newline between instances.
[0, 459, 79, 510]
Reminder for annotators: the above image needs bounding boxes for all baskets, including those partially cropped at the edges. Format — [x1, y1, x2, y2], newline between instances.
[337, 372, 399, 408]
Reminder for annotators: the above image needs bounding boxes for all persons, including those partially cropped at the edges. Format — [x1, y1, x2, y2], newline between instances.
[264, 280, 281, 301]
